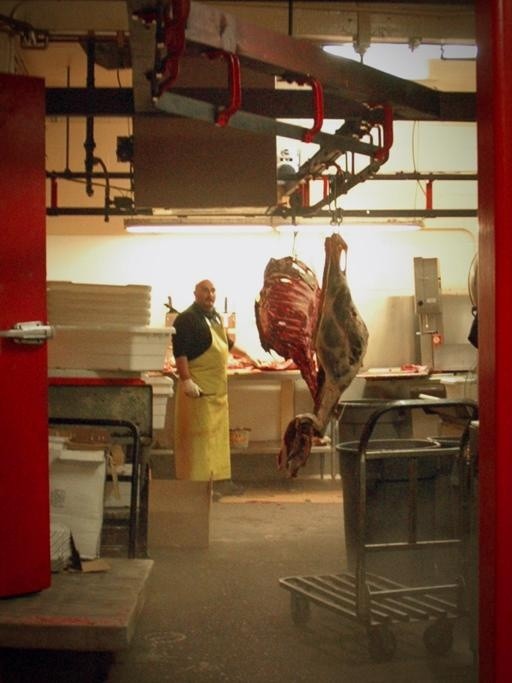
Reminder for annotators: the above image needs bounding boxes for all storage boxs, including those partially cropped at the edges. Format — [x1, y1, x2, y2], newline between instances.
[147, 464, 213, 552]
[47, 281, 151, 325]
[48, 324, 174, 372]
[48, 427, 122, 575]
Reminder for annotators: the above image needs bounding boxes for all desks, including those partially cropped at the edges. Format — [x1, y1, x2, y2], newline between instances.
[151, 368, 334, 479]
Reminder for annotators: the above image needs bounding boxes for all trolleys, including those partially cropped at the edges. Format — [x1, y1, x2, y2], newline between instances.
[276, 398, 478, 663]
[1, 416, 156, 683]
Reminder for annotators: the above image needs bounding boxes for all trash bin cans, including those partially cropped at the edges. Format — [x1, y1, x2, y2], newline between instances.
[335, 437, 461, 581]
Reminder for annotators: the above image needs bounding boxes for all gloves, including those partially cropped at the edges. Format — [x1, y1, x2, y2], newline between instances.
[181, 378, 203, 398]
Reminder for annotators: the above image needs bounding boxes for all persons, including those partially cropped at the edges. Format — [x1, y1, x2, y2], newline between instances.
[172, 279, 258, 498]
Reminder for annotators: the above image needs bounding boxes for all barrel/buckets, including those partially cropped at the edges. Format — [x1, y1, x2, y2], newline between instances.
[362, 376, 447, 401]
[428, 435, 461, 493]
[335, 438, 442, 573]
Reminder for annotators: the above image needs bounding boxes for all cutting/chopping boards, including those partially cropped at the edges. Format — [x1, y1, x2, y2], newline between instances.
[356, 367, 428, 378]
